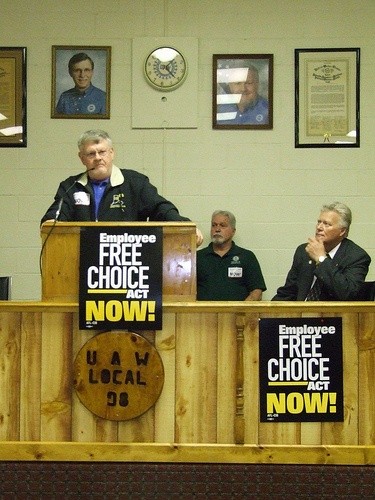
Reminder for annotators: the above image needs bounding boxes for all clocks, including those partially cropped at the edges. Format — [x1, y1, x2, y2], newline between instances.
[130, 36, 200, 129]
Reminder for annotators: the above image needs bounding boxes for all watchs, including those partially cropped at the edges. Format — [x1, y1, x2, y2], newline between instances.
[316, 255, 327, 266]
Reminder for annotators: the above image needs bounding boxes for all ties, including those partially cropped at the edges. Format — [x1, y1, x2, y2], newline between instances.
[306, 254, 331, 301]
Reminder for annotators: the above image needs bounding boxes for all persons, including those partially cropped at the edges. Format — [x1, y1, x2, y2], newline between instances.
[56, 51, 107, 114]
[40, 130, 204, 249]
[271, 201, 371, 304]
[197, 210, 267, 301]
[219, 63, 268, 124]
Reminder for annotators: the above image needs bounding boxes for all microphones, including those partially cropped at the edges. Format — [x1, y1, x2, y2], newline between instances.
[54, 168, 95, 222]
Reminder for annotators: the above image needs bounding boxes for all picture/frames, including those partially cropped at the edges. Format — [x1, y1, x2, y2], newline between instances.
[50, 44, 112, 119]
[213, 53, 274, 130]
